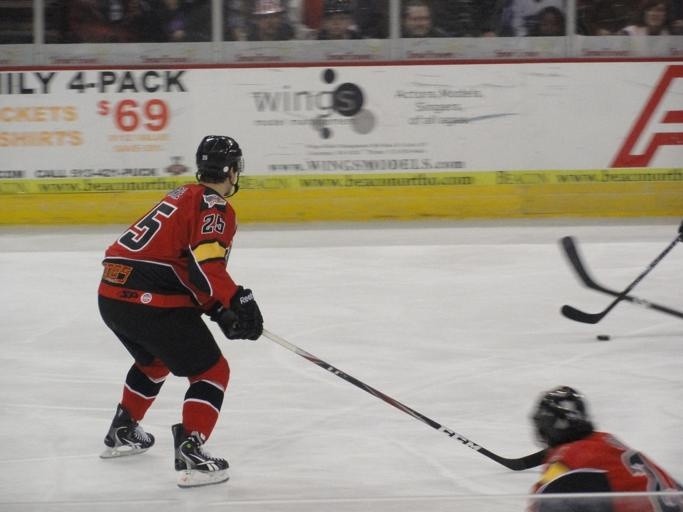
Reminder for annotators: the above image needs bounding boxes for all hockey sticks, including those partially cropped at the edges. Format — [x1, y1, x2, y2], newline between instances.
[263, 330, 551, 472]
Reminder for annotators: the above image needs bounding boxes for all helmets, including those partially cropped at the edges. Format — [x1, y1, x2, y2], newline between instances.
[194, 134, 242, 168]
[530, 384, 587, 441]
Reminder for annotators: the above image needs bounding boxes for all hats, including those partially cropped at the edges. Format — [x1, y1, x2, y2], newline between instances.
[249, 0, 357, 22]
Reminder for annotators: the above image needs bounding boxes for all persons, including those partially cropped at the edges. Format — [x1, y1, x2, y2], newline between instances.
[524, 384, 683, 511]
[96, 134, 264, 472]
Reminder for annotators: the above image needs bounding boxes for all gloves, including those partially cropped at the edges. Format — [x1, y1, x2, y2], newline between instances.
[228, 285, 262, 342]
[203, 298, 239, 342]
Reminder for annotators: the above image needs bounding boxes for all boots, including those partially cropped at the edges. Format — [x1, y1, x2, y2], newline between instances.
[103, 402, 154, 450]
[170, 423, 230, 473]
